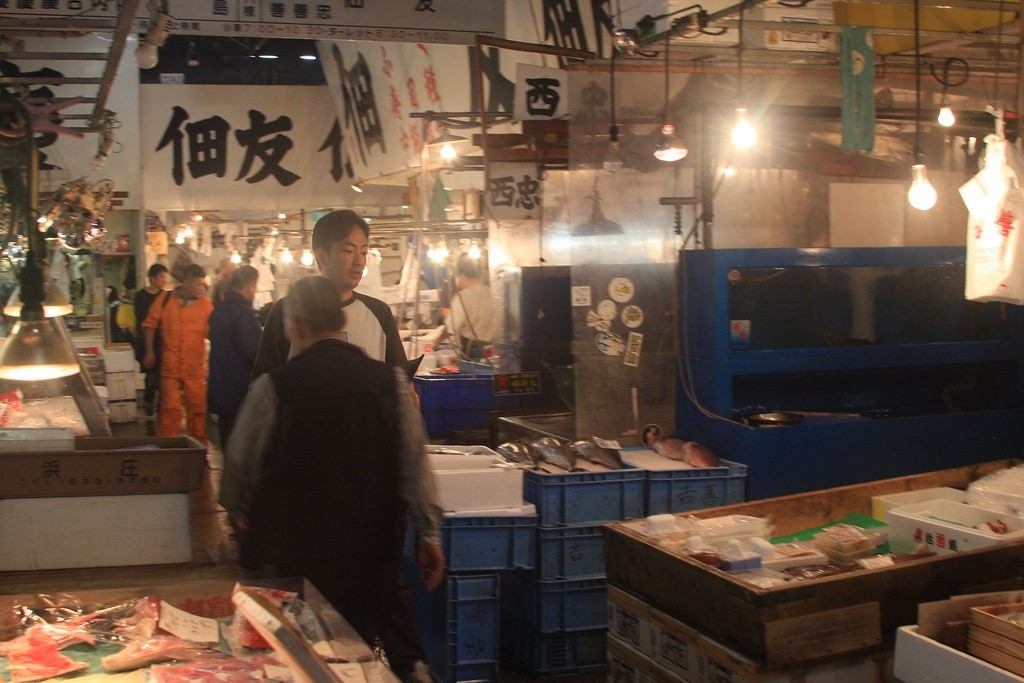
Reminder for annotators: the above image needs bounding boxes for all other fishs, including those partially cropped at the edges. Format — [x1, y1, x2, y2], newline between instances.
[532, 436, 589, 472]
[652, 439, 719, 468]
[567, 439, 634, 469]
[496, 440, 551, 473]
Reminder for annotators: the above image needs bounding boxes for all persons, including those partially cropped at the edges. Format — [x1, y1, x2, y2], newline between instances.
[209, 257, 235, 299]
[207, 266, 273, 445]
[142, 263, 215, 445]
[393, 280, 432, 329]
[217, 277, 443, 650]
[435, 256, 493, 358]
[253, 210, 432, 683]
[134, 264, 168, 416]
[105, 287, 137, 342]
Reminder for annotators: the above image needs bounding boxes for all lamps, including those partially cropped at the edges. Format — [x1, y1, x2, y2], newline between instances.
[0, 97, 83, 383]
[652, 30, 690, 163]
[427, 113, 469, 159]
[566, 58, 625, 237]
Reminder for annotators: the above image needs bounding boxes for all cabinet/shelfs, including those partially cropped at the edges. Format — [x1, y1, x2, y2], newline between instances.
[0, 250, 1024, 683]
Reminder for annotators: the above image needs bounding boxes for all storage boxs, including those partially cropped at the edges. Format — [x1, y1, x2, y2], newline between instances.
[400, 441, 751, 683]
